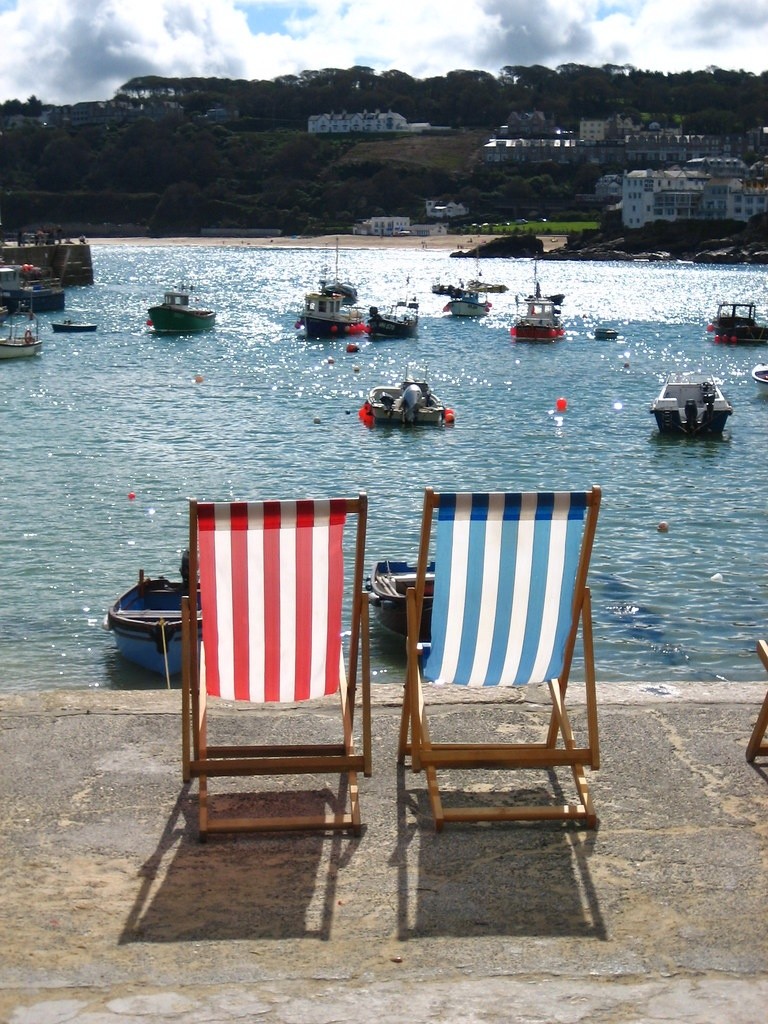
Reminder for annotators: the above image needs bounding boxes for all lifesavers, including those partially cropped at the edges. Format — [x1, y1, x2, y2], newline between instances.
[24, 329, 32, 344]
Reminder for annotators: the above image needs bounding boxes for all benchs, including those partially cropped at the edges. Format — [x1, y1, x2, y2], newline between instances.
[117, 609, 201, 619]
[375, 572, 435, 582]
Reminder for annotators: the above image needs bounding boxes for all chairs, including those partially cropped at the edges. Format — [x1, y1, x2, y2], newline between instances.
[180, 496, 373, 842]
[398, 484, 602, 833]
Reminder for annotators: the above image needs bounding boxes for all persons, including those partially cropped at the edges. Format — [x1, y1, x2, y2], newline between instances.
[459, 278, 464, 288]
[79, 234, 85, 244]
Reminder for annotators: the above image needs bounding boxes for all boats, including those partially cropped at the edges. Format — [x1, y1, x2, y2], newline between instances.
[0, 290, 43, 360]
[712, 300, 768, 343]
[447, 289, 488, 318]
[650, 372, 734, 432]
[49, 318, 98, 333]
[366, 273, 419, 340]
[465, 234, 509, 294]
[510, 249, 567, 344]
[365, 560, 435, 661]
[366, 363, 445, 423]
[297, 290, 366, 336]
[594, 328, 619, 339]
[146, 285, 217, 334]
[0, 222, 65, 315]
[101, 568, 203, 678]
[320, 236, 359, 305]
[751, 363, 768, 383]
[432, 284, 467, 298]
